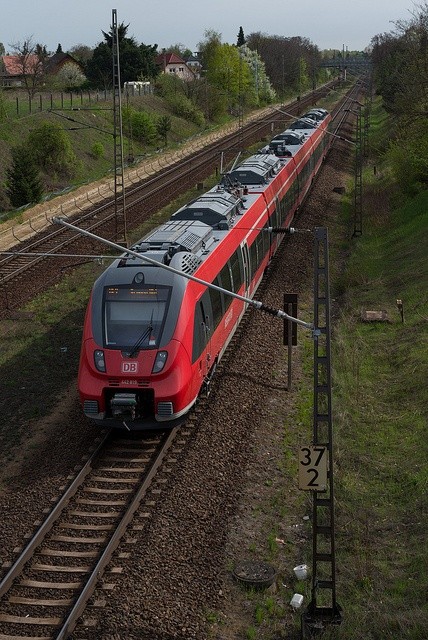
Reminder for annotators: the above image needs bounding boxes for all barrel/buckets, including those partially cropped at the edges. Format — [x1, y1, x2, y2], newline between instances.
[290, 593, 303, 610]
[294, 564, 309, 580]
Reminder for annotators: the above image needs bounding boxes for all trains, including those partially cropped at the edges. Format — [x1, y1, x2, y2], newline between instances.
[77, 108, 333, 430]
